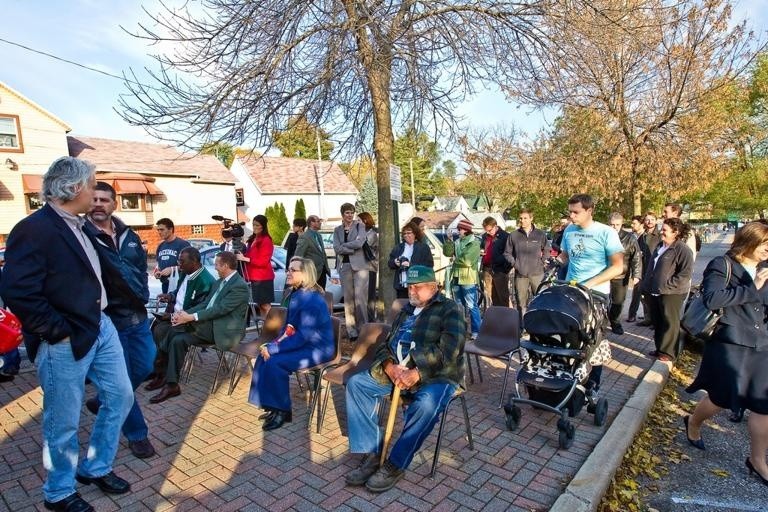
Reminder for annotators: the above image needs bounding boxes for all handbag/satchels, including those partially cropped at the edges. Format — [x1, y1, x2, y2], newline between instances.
[356, 223, 375, 261]
[679, 255, 733, 341]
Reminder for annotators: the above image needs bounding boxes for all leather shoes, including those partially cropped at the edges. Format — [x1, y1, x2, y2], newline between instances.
[729, 410, 744, 424]
[257, 408, 274, 421]
[43, 494, 95, 512]
[86, 374, 181, 458]
[262, 408, 292, 430]
[611, 313, 655, 335]
[345, 452, 387, 486]
[364, 459, 406, 492]
[76, 471, 130, 494]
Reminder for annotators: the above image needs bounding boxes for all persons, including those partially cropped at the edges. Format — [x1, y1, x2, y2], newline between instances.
[727, 219, 767, 423]
[0, 286, 22, 382]
[345, 264, 467, 493]
[81, 181, 158, 459]
[141, 202, 380, 404]
[1, 156, 135, 511]
[544, 192, 625, 407]
[388, 203, 699, 362]
[247, 255, 336, 431]
[682, 221, 768, 488]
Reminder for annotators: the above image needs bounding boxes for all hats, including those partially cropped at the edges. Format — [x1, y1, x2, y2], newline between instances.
[402, 264, 435, 283]
[457, 218, 473, 231]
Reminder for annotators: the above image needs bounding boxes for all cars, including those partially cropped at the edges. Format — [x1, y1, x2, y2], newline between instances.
[174, 225, 483, 311]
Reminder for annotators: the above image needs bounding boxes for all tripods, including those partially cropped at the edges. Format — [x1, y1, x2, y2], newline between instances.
[230, 247, 262, 337]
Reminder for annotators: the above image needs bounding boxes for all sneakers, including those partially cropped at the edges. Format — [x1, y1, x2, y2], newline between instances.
[648, 349, 673, 361]
[585, 388, 598, 402]
[470, 335, 477, 339]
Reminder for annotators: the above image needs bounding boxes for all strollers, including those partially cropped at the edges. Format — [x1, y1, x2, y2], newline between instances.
[504, 280, 611, 439]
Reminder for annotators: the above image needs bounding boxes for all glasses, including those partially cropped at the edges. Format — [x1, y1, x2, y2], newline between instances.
[285, 269, 301, 273]
[486, 228, 493, 234]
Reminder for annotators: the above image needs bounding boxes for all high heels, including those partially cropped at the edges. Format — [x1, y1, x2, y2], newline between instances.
[745, 457, 768, 485]
[682, 414, 706, 451]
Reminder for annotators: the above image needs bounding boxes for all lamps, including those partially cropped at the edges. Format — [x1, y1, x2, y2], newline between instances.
[6, 158, 13, 170]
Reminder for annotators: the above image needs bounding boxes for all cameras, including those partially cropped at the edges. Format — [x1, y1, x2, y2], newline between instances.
[396, 256, 409, 267]
[540, 256, 564, 284]
